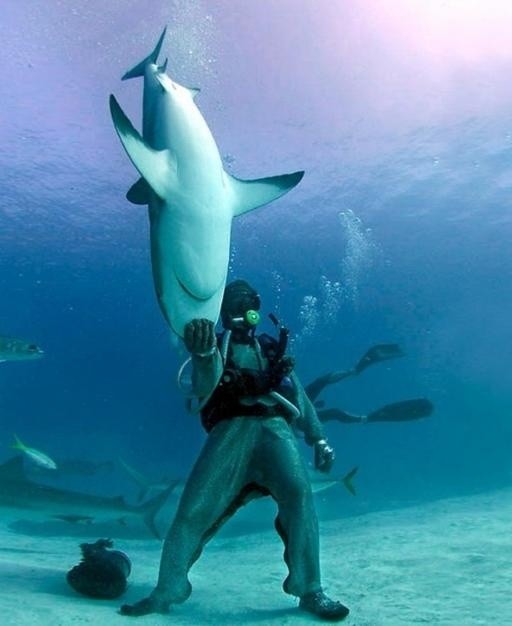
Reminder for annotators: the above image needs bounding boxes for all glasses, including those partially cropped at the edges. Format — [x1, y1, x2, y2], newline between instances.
[222, 294, 261, 315]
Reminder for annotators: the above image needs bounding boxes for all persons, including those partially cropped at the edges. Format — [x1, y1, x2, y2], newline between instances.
[118, 278, 351, 622]
[290, 336, 433, 428]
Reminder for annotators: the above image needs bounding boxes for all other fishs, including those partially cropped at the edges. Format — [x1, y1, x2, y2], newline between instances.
[311, 466, 361, 497]
[1, 333, 45, 363]
[1, 430, 186, 543]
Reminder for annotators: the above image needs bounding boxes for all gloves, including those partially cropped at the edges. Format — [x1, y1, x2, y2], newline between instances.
[184, 317, 219, 358]
[313, 443, 337, 474]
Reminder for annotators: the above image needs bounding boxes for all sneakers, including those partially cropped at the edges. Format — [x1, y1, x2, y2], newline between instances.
[365, 409, 389, 421]
[118, 598, 172, 618]
[353, 356, 370, 377]
[298, 590, 350, 621]
